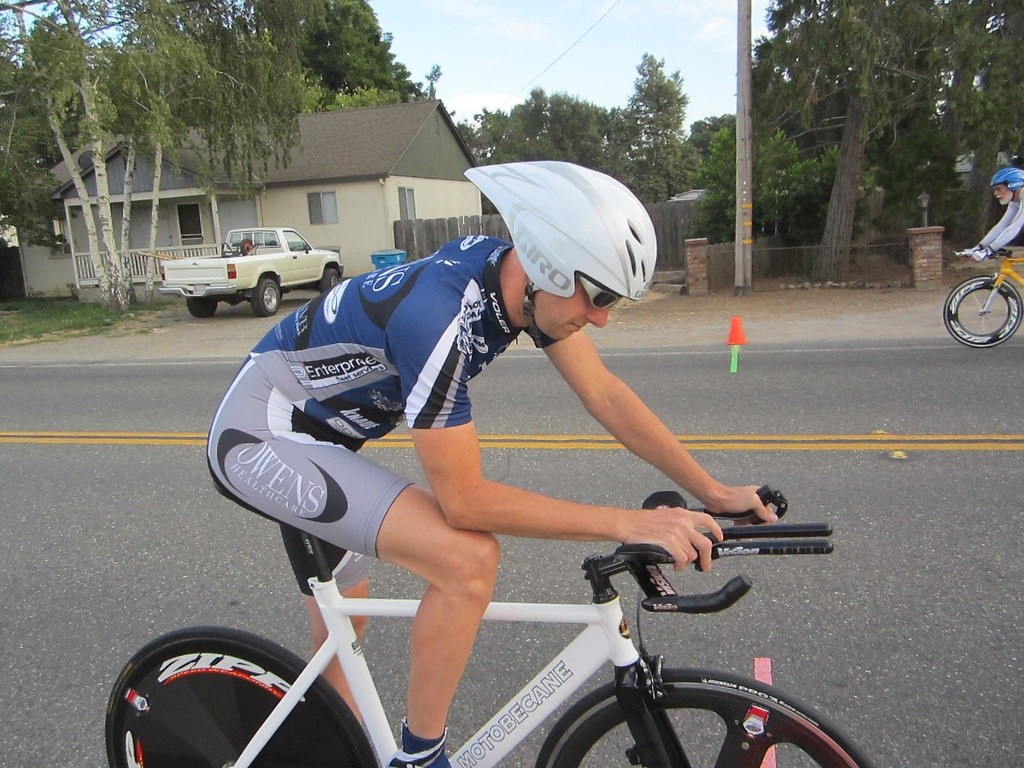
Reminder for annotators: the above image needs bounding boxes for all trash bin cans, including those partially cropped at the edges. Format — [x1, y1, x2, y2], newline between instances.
[369, 248, 407, 271]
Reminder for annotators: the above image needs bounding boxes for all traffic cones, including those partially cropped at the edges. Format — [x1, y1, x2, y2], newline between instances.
[725, 312, 747, 344]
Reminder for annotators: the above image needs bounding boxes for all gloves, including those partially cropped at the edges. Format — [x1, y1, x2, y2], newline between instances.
[963, 244, 981, 257]
[971, 248, 991, 261]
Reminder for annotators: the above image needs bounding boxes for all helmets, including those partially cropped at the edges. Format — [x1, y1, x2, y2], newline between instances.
[463, 160, 658, 301]
[990, 167, 1024, 192]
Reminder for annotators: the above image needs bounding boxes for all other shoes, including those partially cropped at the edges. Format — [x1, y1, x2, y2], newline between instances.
[384, 727, 449, 768]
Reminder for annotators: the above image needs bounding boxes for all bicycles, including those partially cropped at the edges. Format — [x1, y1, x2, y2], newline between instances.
[941, 249, 1023, 350]
[107, 483, 886, 767]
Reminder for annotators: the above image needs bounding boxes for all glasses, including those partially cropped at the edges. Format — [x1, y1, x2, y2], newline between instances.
[576, 274, 622, 310]
[992, 186, 1009, 191]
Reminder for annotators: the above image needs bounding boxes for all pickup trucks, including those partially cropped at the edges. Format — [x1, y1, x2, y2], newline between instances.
[158, 226, 346, 318]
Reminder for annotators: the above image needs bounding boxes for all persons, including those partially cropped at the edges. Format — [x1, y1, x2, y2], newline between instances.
[206, 159, 778, 768]
[963, 167, 1024, 261]
